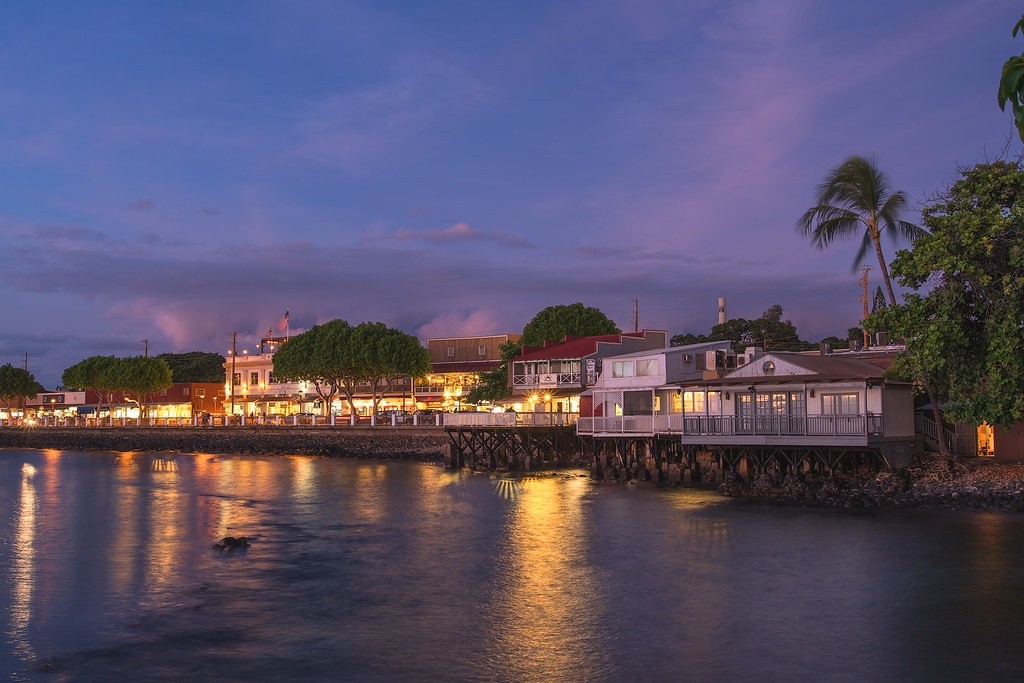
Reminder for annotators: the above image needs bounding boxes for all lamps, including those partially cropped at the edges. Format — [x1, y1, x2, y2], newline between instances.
[810, 389, 814, 398]
[51, 399, 55, 403]
[725, 391, 730, 400]
[677, 389, 681, 395]
[39, 406, 44, 411]
[868, 383, 873, 390]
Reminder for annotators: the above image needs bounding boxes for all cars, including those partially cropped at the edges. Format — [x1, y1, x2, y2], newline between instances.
[253, 413, 315, 425]
[11, 414, 86, 427]
[370, 408, 445, 427]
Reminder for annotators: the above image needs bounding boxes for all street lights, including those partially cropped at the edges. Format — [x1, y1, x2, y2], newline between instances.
[21, 352, 28, 370]
[140, 339, 147, 357]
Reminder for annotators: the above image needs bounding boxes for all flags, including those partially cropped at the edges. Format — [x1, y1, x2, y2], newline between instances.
[278, 310, 289, 334]
[265, 327, 272, 338]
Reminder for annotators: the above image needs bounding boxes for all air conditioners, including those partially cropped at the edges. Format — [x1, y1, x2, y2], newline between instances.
[682, 354, 693, 361]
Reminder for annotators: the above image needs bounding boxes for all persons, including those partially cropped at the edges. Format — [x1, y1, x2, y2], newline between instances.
[202, 412, 211, 426]
[262, 412, 266, 423]
[250, 411, 254, 424]
